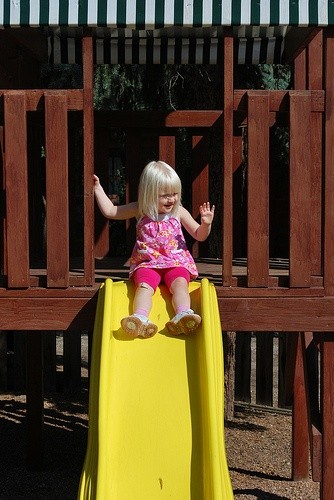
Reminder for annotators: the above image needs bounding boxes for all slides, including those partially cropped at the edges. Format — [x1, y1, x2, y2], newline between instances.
[77, 277, 237, 500]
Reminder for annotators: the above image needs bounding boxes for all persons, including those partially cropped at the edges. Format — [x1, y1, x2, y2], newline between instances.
[92, 160, 217, 337]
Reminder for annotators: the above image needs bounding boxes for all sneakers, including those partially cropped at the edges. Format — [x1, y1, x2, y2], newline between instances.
[120, 314, 158, 338]
[166, 311, 201, 334]
